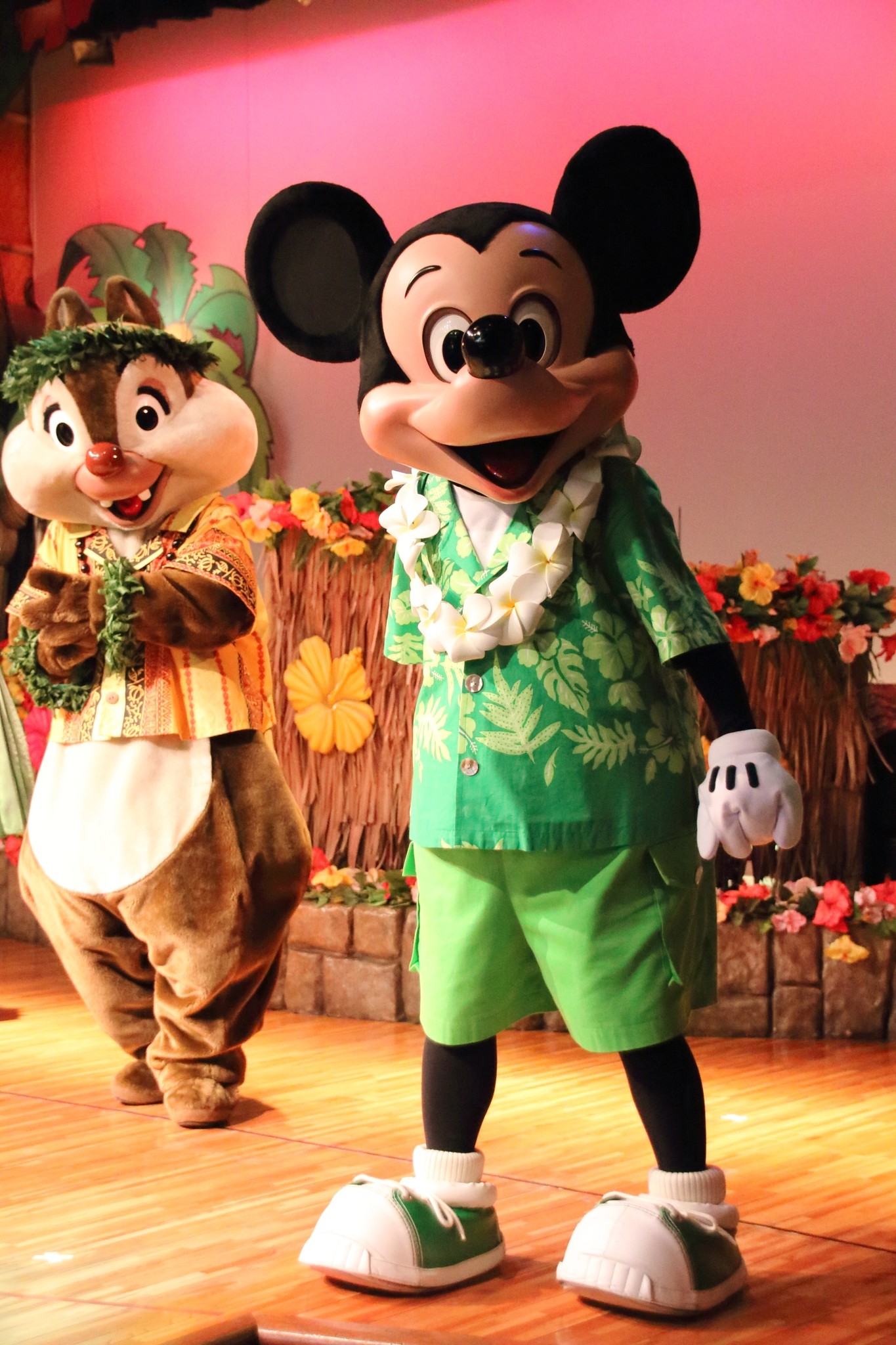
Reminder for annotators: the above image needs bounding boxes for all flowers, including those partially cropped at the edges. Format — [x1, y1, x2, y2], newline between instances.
[223, 470, 896, 965]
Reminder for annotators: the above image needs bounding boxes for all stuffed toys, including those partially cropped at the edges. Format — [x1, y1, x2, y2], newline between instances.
[0, 277, 312, 1127]
[243, 125, 804, 1320]
